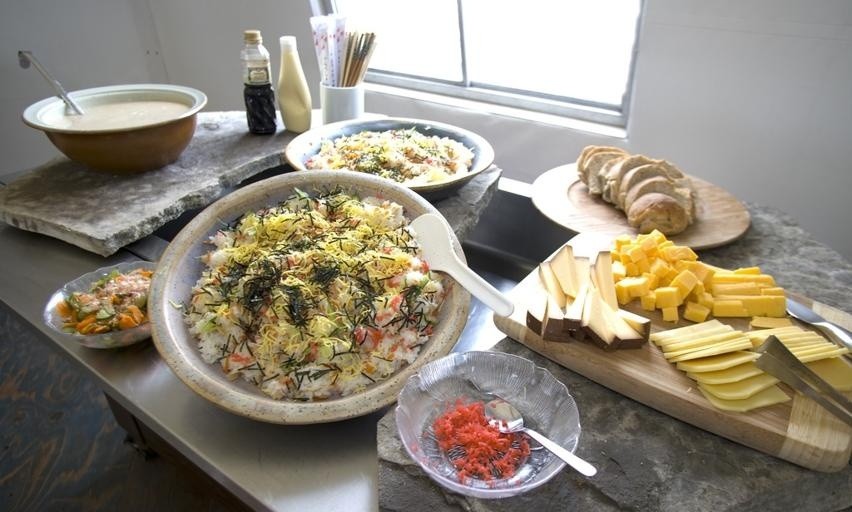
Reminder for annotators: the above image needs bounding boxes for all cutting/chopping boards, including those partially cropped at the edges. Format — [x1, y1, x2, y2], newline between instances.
[493, 229, 852, 476]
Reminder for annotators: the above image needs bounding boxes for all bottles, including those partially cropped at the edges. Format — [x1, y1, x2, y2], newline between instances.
[240, 30, 312, 134]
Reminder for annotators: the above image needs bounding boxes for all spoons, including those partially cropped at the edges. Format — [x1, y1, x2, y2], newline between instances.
[481, 398, 599, 482]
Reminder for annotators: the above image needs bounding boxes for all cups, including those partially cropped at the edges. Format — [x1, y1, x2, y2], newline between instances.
[320, 82, 364, 124]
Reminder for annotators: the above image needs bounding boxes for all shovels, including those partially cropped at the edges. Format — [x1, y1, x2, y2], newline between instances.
[409, 214, 514, 318]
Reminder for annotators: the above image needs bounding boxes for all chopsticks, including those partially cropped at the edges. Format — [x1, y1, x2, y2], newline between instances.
[341, 31, 377, 89]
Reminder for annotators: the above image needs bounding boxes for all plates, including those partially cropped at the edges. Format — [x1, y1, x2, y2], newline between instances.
[394, 350, 579, 499]
[532, 160, 751, 254]
[42, 260, 158, 352]
[287, 119, 497, 207]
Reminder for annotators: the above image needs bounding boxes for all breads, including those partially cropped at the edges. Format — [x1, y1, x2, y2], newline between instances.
[575, 144, 706, 236]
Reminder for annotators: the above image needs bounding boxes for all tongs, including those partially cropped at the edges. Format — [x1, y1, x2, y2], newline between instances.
[756, 334, 852, 430]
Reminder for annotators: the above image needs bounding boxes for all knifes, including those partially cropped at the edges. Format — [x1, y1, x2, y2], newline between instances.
[784, 298, 852, 358]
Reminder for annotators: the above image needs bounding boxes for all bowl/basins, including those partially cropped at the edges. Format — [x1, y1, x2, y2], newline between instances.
[146, 170, 473, 429]
[22, 82, 209, 172]
